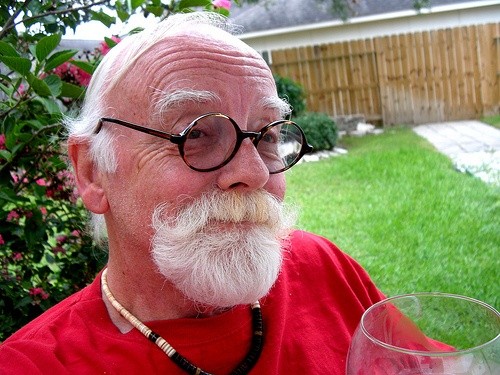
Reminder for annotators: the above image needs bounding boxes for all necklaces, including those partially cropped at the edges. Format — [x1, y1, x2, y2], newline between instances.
[98, 265, 267, 375]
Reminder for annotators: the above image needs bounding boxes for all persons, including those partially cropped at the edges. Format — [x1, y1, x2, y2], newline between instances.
[0, 15, 478, 374]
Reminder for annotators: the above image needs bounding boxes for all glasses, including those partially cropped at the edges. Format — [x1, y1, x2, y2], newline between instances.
[94, 112, 314, 176]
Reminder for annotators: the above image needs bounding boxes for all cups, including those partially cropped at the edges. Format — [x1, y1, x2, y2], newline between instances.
[346, 291, 500, 375]
